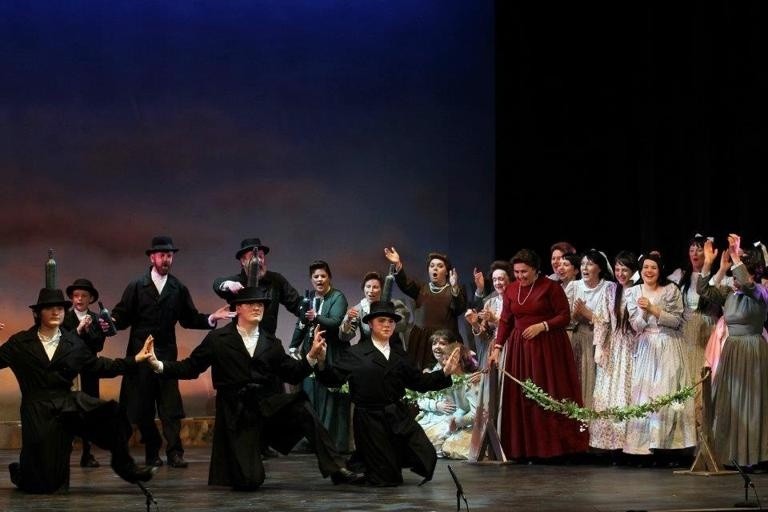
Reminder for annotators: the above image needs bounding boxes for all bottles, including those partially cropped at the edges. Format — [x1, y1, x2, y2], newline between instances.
[246, 246, 260, 288]
[301, 289, 311, 325]
[97, 300, 116, 336]
[44, 247, 57, 292]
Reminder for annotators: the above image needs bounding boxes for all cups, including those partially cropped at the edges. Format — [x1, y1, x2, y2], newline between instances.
[351, 307, 360, 322]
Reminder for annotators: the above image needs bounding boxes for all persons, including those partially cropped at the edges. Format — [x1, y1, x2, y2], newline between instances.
[287, 245, 477, 487]
[0, 233, 768, 494]
[465, 234, 767, 469]
[1, 232, 364, 492]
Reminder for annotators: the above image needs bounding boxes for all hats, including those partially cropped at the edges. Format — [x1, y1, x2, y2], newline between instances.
[236, 238, 270, 259]
[29, 288, 72, 311]
[66, 279, 99, 304]
[145, 236, 179, 256]
[227, 287, 272, 304]
[362, 301, 402, 323]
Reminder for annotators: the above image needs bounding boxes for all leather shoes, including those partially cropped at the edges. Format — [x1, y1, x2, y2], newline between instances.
[167, 451, 188, 467]
[124, 465, 158, 484]
[330, 468, 365, 485]
[81, 453, 100, 467]
[145, 455, 163, 466]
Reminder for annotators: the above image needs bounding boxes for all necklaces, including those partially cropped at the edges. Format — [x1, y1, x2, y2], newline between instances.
[429, 282, 450, 294]
[517, 281, 534, 305]
